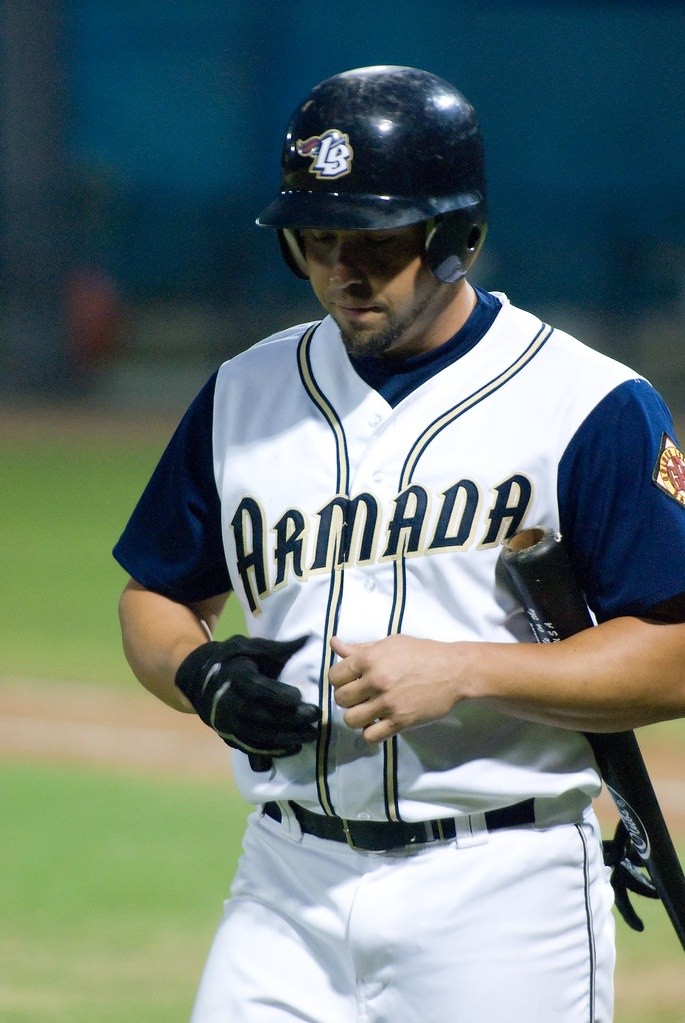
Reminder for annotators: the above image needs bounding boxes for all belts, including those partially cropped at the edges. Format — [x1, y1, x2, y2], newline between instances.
[262, 795, 535, 850]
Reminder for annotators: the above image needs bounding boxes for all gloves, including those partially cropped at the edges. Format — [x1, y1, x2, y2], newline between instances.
[175, 633, 323, 773]
[602, 818, 661, 932]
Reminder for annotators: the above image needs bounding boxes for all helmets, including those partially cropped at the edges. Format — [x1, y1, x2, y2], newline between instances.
[254, 65, 488, 284]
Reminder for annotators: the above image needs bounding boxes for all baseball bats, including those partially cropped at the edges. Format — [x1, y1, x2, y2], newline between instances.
[500, 526, 685, 957]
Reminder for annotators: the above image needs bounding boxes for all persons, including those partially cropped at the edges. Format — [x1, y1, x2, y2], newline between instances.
[109, 65, 685, 1023]
